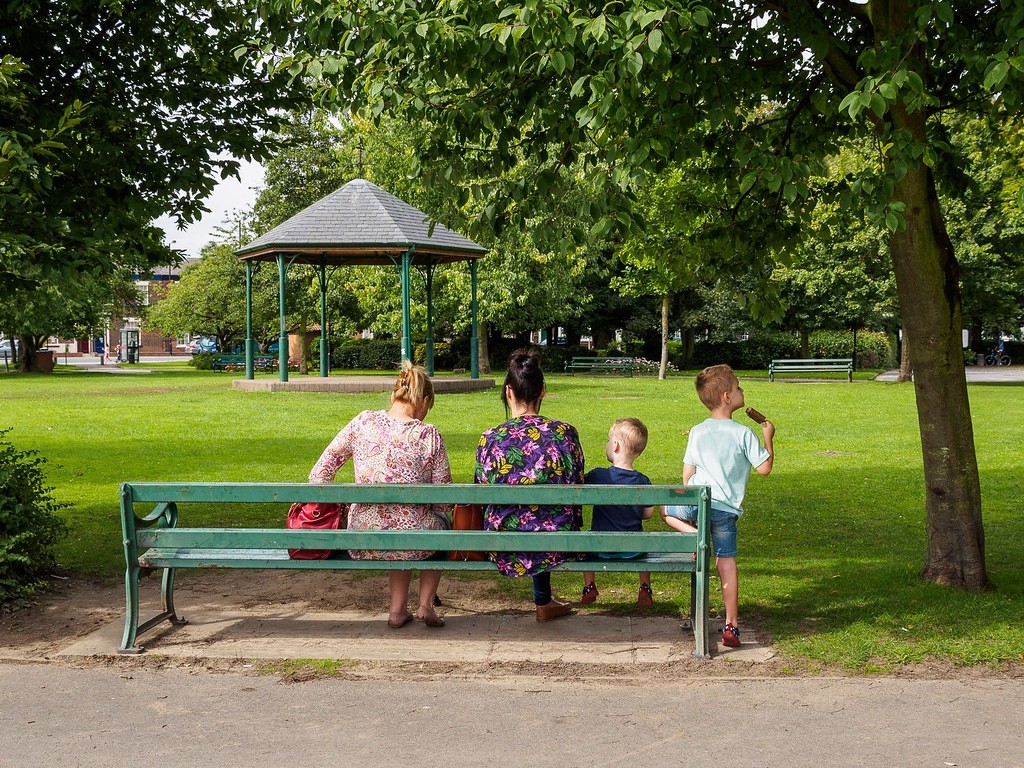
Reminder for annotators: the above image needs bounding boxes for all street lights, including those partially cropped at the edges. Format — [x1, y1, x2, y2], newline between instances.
[235, 220, 242, 248]
[169, 240, 176, 355]
[845, 318, 863, 371]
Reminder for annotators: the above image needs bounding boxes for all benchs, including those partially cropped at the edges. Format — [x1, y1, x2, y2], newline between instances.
[769, 359, 853, 382]
[120, 481, 713, 652]
[213, 355, 274, 375]
[565, 357, 637, 376]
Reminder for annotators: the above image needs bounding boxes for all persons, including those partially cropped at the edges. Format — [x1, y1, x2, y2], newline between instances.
[114, 345, 124, 362]
[659, 364, 775, 647]
[308, 359, 456, 627]
[994, 339, 1004, 366]
[102, 343, 112, 363]
[579, 417, 657, 608]
[474, 344, 586, 622]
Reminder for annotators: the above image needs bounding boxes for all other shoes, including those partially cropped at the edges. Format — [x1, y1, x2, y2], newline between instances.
[536, 602, 571, 623]
[582, 583, 598, 604]
[415, 605, 445, 626]
[638, 583, 652, 607]
[692, 553, 698, 559]
[722, 623, 740, 647]
[388, 612, 413, 628]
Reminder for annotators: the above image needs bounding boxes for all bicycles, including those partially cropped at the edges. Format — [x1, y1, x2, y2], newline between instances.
[984, 349, 1012, 367]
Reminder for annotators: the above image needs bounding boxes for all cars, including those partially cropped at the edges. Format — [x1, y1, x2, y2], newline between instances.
[539, 336, 567, 345]
[0, 340, 19, 357]
[185, 338, 201, 355]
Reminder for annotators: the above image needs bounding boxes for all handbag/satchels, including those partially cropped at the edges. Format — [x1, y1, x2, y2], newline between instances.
[285, 502, 349, 559]
[451, 504, 484, 561]
